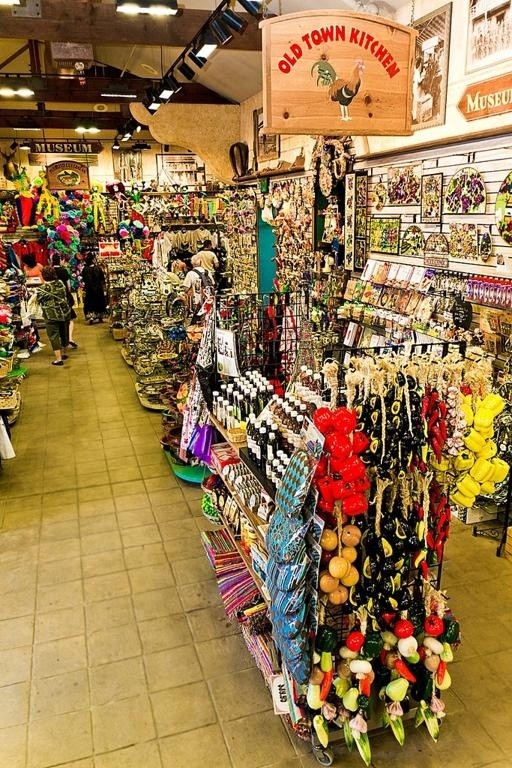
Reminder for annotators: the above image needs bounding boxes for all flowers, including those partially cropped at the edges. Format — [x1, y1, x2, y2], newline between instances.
[37, 215, 79, 259]
[16, 169, 43, 199]
[59, 191, 93, 235]
[118, 219, 137, 242]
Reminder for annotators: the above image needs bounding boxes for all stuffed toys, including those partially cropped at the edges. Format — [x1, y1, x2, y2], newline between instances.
[88, 184, 112, 235]
[125, 180, 146, 214]
[106, 181, 127, 205]
[32, 171, 61, 218]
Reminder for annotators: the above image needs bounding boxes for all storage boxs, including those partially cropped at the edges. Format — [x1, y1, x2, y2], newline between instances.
[111, 322, 124, 341]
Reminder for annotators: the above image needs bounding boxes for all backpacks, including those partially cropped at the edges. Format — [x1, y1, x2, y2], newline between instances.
[190, 269, 216, 296]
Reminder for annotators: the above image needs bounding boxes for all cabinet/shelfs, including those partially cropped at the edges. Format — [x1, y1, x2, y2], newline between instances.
[225, 176, 314, 291]
[338, 259, 511, 558]
[201, 341, 467, 762]
[2, 189, 122, 308]
[139, 190, 232, 298]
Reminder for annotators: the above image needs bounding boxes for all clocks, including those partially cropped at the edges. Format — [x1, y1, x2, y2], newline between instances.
[134, 354, 155, 376]
[452, 301, 473, 329]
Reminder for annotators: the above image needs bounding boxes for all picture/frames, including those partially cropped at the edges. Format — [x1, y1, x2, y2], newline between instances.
[494, 171, 512, 247]
[156, 153, 207, 187]
[420, 172, 443, 224]
[252, 106, 281, 163]
[425, 231, 450, 256]
[442, 168, 487, 215]
[462, 0, 511, 79]
[369, 217, 401, 255]
[406, 0, 453, 132]
[480, 231, 493, 263]
[401, 225, 425, 260]
[448, 220, 479, 262]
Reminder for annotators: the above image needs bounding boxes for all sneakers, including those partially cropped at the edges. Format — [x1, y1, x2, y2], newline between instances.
[90, 318, 94, 325]
[70, 340, 78, 348]
[62, 354, 69, 359]
[52, 361, 64, 367]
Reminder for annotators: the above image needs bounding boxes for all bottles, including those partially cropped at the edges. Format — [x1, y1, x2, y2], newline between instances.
[293, 415, 303, 451]
[226, 388, 232, 405]
[212, 391, 219, 417]
[246, 413, 255, 430]
[249, 392, 258, 419]
[220, 384, 226, 400]
[279, 402, 289, 440]
[275, 465, 283, 492]
[226, 405, 234, 429]
[258, 386, 267, 413]
[244, 389, 250, 417]
[269, 424, 279, 447]
[222, 400, 227, 429]
[284, 391, 290, 402]
[227, 369, 270, 396]
[251, 421, 261, 462]
[276, 449, 291, 469]
[216, 397, 223, 423]
[282, 407, 292, 449]
[288, 395, 296, 407]
[236, 394, 244, 422]
[287, 411, 298, 455]
[269, 393, 278, 419]
[256, 427, 267, 468]
[266, 384, 273, 403]
[271, 460, 280, 489]
[247, 418, 257, 457]
[265, 453, 273, 480]
[298, 403, 310, 421]
[267, 433, 277, 461]
[292, 400, 301, 411]
[232, 391, 239, 419]
[274, 398, 283, 426]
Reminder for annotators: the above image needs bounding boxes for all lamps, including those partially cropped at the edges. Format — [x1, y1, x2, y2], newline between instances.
[100, 79, 137, 99]
[120, 125, 133, 141]
[110, 147, 144, 187]
[9, 138, 18, 150]
[155, 80, 172, 105]
[194, 26, 219, 59]
[131, 139, 151, 153]
[187, 39, 207, 69]
[166, 68, 183, 93]
[207, 12, 233, 46]
[0, 73, 36, 99]
[176, 54, 195, 81]
[142, 88, 162, 116]
[116, 1, 185, 18]
[112, 137, 120, 150]
[73, 115, 102, 136]
[20, 138, 32, 151]
[238, 0, 263, 14]
[13, 115, 41, 131]
[156, 80, 174, 99]
[219, 0, 249, 34]
[129, 119, 141, 133]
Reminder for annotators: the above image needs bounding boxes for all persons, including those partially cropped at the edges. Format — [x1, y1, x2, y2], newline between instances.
[82, 255, 107, 324]
[33, 267, 70, 366]
[20, 253, 43, 275]
[198, 240, 218, 276]
[53, 253, 82, 348]
[170, 252, 187, 278]
[184, 256, 214, 306]
